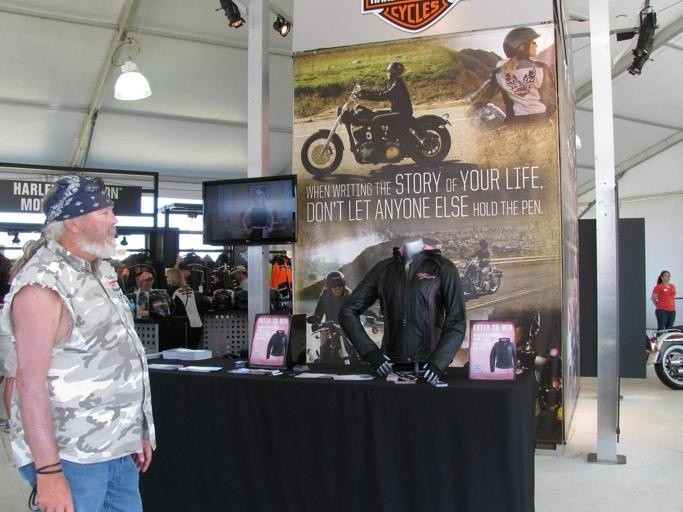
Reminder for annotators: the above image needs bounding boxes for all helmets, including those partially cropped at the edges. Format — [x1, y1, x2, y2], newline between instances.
[327, 271, 344, 287]
[503, 27, 539, 57]
[386, 62, 404, 75]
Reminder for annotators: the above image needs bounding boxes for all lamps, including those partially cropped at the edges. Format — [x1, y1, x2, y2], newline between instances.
[215, 0, 246, 28]
[272, 14, 291, 37]
[121, 234, 128, 246]
[12, 232, 20, 243]
[616, 0, 661, 77]
[111, 33, 152, 101]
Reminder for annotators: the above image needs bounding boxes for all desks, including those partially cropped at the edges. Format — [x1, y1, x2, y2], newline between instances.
[138, 354, 536, 512]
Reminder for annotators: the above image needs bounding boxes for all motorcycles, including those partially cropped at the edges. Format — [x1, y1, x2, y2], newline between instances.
[301, 82, 453, 178]
[307, 317, 384, 364]
[458, 254, 503, 296]
[645, 325, 683, 389]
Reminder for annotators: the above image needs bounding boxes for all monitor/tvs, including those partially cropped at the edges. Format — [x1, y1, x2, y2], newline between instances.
[202, 174, 298, 246]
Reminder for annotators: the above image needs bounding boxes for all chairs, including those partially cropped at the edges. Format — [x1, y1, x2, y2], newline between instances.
[113, 253, 292, 350]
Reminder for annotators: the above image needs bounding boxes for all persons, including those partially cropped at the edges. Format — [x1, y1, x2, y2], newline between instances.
[241, 188, 274, 240]
[468, 239, 491, 288]
[307, 271, 369, 366]
[652, 270, 677, 340]
[118, 267, 250, 350]
[0, 174, 158, 512]
[488, 306, 561, 441]
[338, 236, 467, 383]
[355, 61, 414, 160]
[463, 26, 557, 134]
[0, 254, 18, 434]
